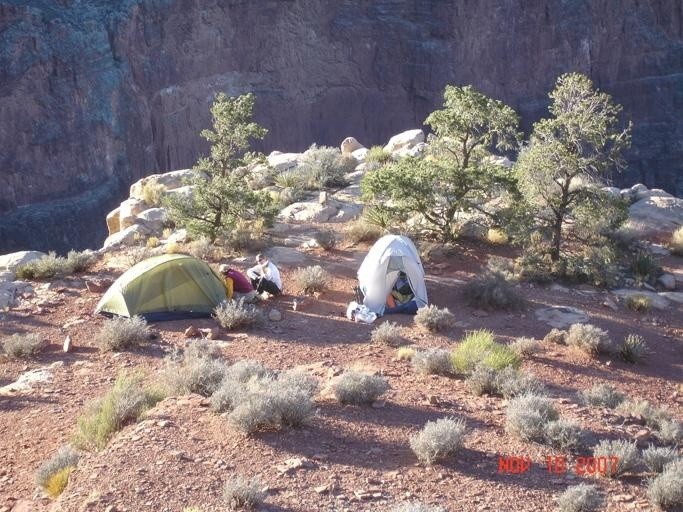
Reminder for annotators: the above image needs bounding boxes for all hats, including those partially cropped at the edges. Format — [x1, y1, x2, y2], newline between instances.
[220, 264, 230, 272]
[256, 254, 265, 261]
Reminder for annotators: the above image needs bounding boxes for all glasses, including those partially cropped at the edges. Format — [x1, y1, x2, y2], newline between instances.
[259, 259, 264, 261]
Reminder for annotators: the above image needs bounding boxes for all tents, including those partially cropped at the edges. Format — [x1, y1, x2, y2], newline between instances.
[95, 252, 227, 323]
[356, 233, 428, 316]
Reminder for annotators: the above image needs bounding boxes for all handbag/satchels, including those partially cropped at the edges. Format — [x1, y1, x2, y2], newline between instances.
[399, 283, 411, 295]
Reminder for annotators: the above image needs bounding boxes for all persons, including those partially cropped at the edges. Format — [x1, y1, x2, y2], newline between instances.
[218, 263, 255, 302]
[246, 253, 281, 295]
